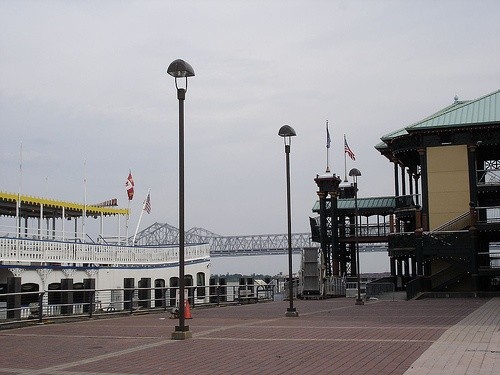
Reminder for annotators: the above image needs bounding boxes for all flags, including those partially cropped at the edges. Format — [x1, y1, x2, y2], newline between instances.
[143, 191, 152, 214]
[326, 127, 331, 148]
[125, 173, 135, 201]
[344, 139, 356, 161]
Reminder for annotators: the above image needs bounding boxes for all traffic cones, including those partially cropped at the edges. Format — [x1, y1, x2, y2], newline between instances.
[184, 300, 193, 319]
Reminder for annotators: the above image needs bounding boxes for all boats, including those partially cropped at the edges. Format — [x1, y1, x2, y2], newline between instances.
[0, 193, 214, 318]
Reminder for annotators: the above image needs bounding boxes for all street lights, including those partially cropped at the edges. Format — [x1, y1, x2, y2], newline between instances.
[277, 124, 297, 312]
[349, 167, 363, 302]
[167, 58, 196, 331]
